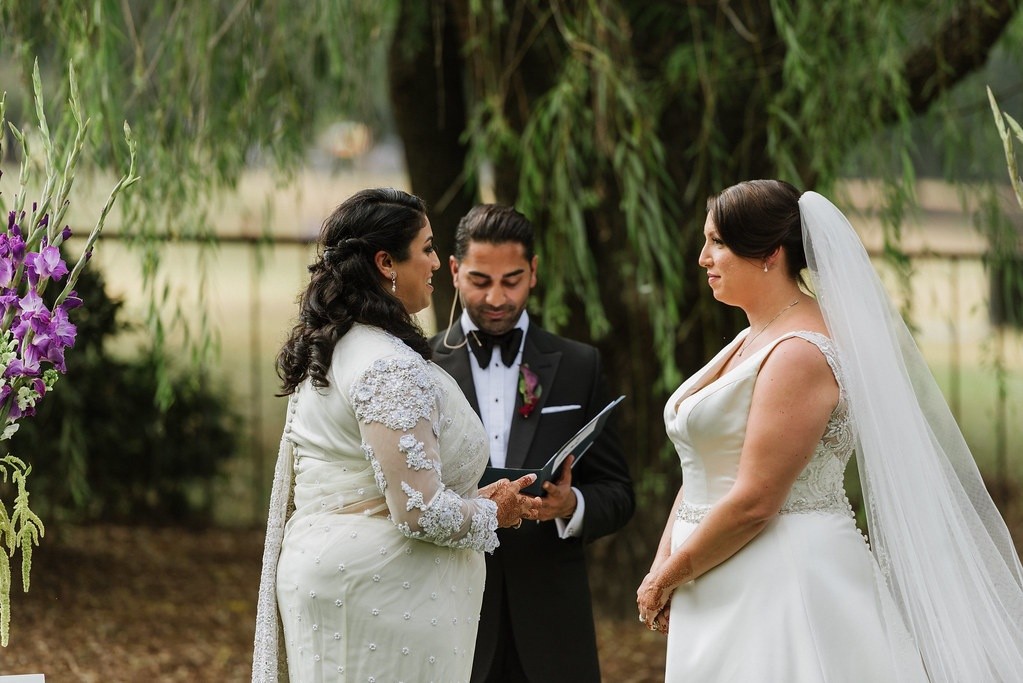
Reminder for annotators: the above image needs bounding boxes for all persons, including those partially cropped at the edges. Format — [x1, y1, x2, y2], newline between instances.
[253, 188, 553, 683]
[636, 178, 1021, 682]
[424, 203, 636, 683]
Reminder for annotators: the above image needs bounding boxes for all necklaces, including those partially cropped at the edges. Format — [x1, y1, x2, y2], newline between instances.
[738, 301, 798, 356]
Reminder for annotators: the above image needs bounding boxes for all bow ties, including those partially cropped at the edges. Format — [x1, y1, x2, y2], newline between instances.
[467, 328, 523, 370]
